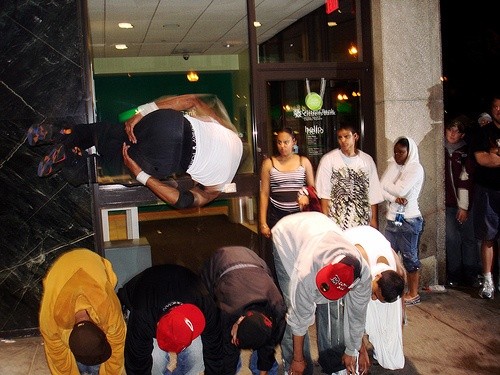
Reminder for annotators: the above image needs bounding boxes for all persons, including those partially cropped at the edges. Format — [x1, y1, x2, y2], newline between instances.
[342, 225, 407, 374]
[378, 136, 426, 307]
[39, 248, 128, 375]
[125, 263, 221, 375]
[258, 128, 315, 291]
[203, 247, 286, 374]
[269, 211, 372, 375]
[315, 124, 385, 236]
[470, 94, 500, 302]
[26, 95, 244, 210]
[477, 111, 491, 128]
[442, 120, 470, 287]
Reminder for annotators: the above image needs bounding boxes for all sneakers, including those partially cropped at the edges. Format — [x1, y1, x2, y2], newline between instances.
[403, 294, 421, 304]
[35, 144, 70, 177]
[27, 121, 57, 146]
[480, 272, 495, 301]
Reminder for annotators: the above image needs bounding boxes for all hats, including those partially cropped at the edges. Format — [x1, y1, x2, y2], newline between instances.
[156, 304, 205, 355]
[236, 307, 273, 349]
[67, 320, 112, 366]
[446, 120, 465, 133]
[315, 263, 354, 300]
